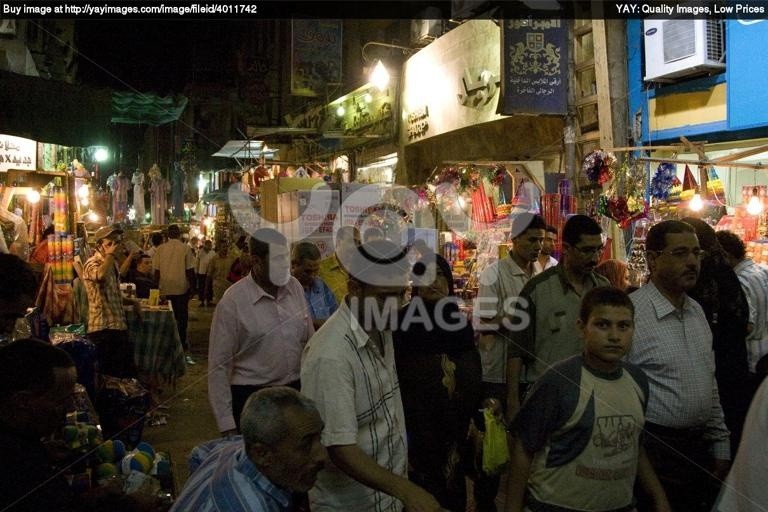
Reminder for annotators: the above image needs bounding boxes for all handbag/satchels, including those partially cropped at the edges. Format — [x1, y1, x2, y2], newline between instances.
[48, 324, 95, 379]
[97, 376, 150, 451]
[482, 408, 512, 475]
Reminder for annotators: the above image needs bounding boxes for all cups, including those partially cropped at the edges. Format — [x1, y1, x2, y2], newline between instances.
[150, 289, 160, 306]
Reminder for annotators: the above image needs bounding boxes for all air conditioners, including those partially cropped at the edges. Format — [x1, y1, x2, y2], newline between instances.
[642, 16, 725, 84]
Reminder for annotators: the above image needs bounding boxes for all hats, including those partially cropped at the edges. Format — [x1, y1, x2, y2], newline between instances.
[95, 226, 123, 243]
[682, 165, 698, 193]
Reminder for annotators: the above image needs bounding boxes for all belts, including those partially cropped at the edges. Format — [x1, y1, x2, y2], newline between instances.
[644, 420, 708, 439]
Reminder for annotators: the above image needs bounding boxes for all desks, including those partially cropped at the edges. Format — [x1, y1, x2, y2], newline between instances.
[128, 300, 180, 389]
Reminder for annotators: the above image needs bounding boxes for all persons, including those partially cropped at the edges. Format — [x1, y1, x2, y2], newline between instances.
[624, 220, 733, 512]
[4, 214, 767, 511]
[302, 238, 447, 511]
[206, 225, 312, 435]
[0, 248, 78, 511]
[500, 284, 670, 511]
[168, 384, 325, 511]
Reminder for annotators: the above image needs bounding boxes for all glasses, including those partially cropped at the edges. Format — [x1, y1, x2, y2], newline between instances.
[573, 244, 604, 253]
[657, 248, 705, 261]
